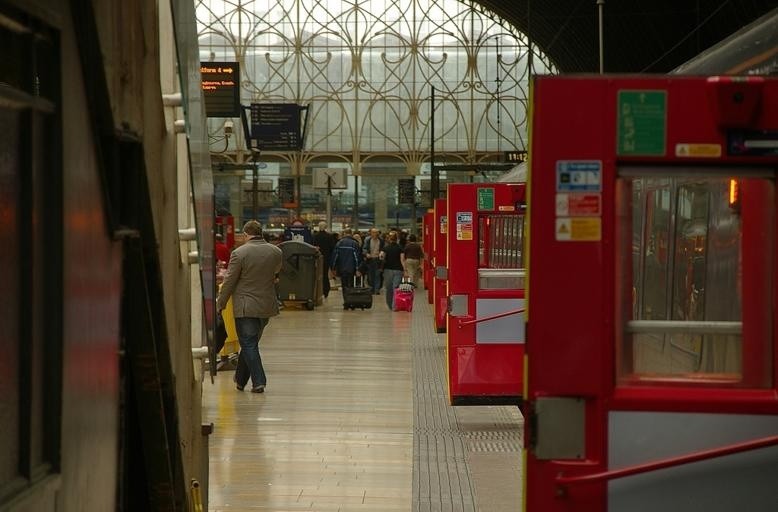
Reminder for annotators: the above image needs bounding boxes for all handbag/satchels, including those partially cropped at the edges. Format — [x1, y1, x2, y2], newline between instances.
[215, 312, 228, 353]
[399, 282, 418, 292]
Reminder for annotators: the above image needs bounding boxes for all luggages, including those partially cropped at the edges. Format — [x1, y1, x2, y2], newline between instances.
[343, 274, 372, 309]
[394, 275, 413, 311]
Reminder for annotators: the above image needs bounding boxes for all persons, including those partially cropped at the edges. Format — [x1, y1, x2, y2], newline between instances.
[215, 218, 283, 393]
[215, 234, 230, 269]
[314, 221, 424, 311]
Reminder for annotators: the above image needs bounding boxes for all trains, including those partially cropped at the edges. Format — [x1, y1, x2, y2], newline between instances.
[478, 6, 778, 383]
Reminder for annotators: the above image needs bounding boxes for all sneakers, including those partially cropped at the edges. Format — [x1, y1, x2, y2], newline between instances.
[232, 376, 264, 392]
